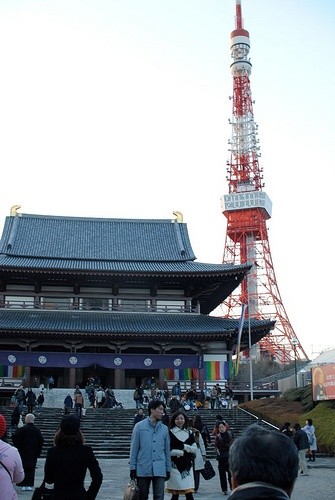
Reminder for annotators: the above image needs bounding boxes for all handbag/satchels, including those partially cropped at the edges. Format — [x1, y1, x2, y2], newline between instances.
[199, 460, 216, 480]
[30, 479, 58, 499]
[124, 475, 141, 499]
[82, 408, 86, 415]
[17, 419, 23, 429]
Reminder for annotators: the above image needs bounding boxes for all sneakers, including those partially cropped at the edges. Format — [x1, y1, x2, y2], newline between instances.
[222, 491, 228, 495]
[301, 473, 309, 476]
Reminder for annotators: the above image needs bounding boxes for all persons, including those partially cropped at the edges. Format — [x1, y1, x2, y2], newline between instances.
[314, 372, 327, 399]
[226, 423, 299, 499]
[12, 413, 43, 490]
[166, 412, 197, 500]
[280, 422, 293, 438]
[293, 423, 310, 476]
[44, 414, 103, 500]
[215, 422, 234, 495]
[187, 426, 206, 493]
[301, 419, 317, 462]
[0, 375, 235, 449]
[0, 413, 24, 500]
[129, 400, 171, 500]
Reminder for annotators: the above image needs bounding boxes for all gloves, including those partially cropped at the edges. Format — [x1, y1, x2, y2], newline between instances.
[130, 470, 136, 480]
[165, 471, 171, 481]
